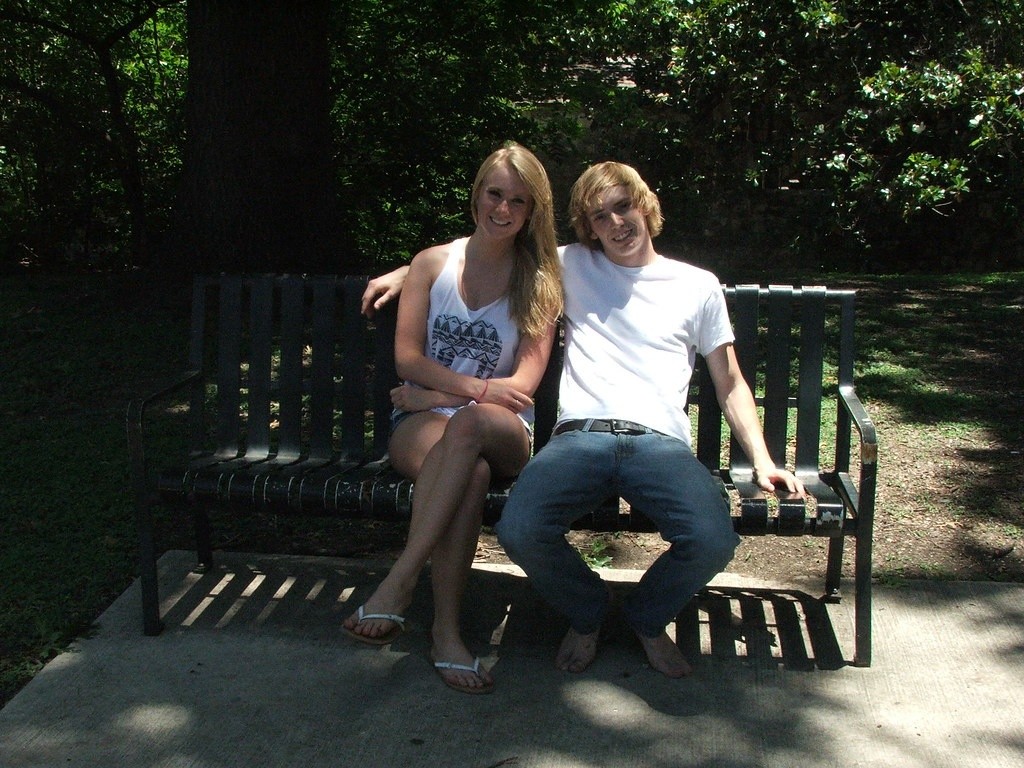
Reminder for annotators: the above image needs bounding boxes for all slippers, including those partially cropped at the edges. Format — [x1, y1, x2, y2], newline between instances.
[339, 605, 412, 646]
[426, 645, 496, 694]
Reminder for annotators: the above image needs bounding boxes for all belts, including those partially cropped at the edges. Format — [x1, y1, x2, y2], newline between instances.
[555, 418, 647, 435]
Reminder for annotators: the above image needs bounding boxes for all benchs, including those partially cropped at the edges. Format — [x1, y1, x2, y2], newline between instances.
[128, 274, 877, 667]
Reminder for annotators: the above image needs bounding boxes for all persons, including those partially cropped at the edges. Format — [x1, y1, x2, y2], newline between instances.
[360, 162, 808, 677]
[341, 144, 566, 691]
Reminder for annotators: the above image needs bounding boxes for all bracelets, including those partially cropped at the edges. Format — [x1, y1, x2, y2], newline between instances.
[476, 379, 489, 402]
